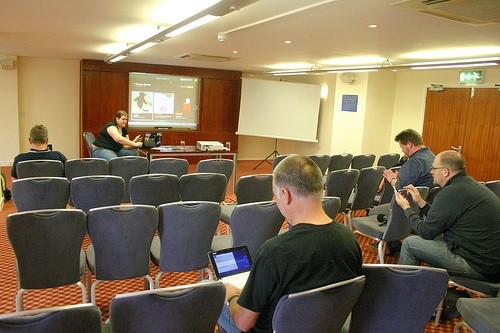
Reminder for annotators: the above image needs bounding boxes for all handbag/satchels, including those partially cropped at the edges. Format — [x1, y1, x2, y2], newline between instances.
[439, 288, 471, 319]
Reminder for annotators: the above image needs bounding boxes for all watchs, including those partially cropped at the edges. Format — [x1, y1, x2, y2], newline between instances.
[227, 295, 240, 309]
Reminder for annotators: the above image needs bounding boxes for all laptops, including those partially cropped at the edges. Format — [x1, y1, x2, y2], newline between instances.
[134, 132, 163, 149]
[207, 245, 252, 289]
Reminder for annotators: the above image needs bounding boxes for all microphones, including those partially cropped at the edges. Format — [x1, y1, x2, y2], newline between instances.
[377, 213, 388, 222]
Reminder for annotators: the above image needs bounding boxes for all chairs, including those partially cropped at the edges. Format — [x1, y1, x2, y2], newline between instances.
[0, 133, 500, 333]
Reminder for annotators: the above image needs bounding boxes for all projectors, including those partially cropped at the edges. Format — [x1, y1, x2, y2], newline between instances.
[197, 140, 223, 151]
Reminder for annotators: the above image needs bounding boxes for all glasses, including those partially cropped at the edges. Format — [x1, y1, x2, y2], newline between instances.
[430, 166, 451, 172]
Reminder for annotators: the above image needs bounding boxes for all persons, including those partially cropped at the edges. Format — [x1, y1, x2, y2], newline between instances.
[395, 149, 500, 317]
[367, 129, 437, 256]
[11, 124, 68, 180]
[90, 110, 144, 160]
[200, 155, 362, 333]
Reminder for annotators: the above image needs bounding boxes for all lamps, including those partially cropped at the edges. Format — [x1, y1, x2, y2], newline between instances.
[264, 57, 500, 76]
[104, 0, 259, 64]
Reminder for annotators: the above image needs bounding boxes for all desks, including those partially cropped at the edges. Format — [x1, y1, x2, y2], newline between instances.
[135, 146, 239, 194]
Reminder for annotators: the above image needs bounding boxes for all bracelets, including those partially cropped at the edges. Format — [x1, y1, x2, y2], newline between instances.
[389, 177, 397, 183]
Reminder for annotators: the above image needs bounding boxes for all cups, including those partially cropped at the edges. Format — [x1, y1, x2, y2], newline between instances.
[226, 142, 230, 151]
[181, 141, 185, 150]
